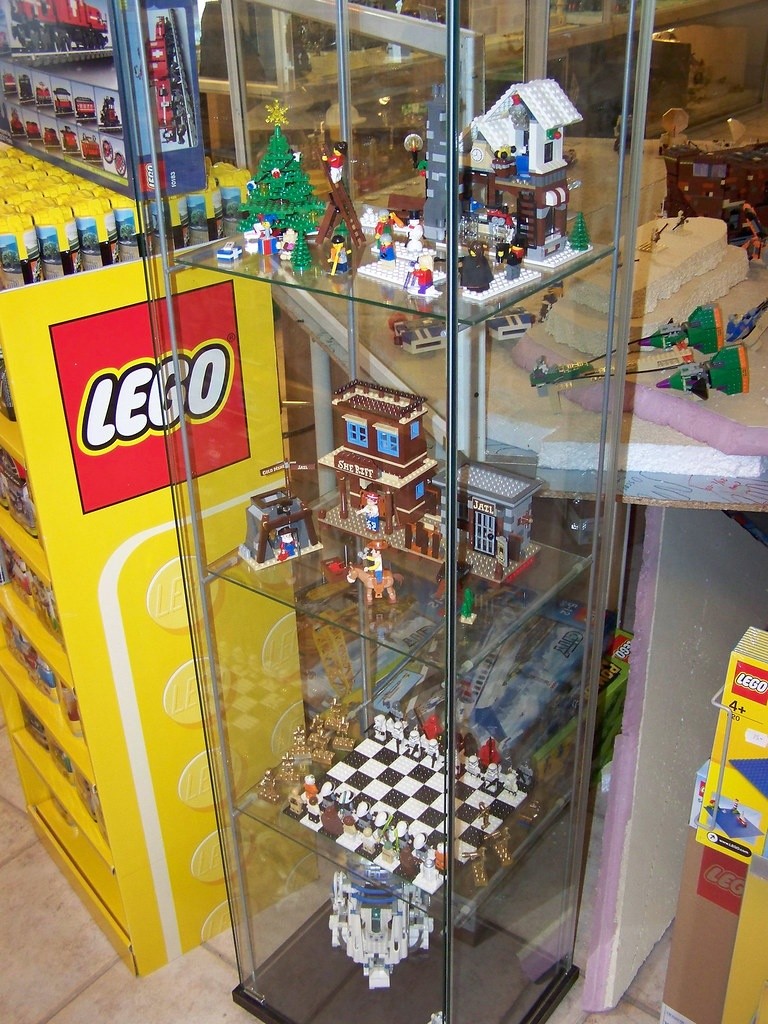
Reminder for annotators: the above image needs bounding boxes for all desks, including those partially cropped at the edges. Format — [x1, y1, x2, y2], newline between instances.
[263, 135, 766, 1012]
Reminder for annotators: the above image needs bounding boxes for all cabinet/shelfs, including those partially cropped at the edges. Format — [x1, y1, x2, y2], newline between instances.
[107, 1, 658, 1023]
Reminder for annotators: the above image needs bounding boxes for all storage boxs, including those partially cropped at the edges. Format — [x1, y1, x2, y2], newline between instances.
[662, 825, 749, 1024]
[0, 0, 209, 202]
[0, 237, 320, 977]
[0, 541, 108, 843]
[0, 349, 16, 422]
[0, 446, 39, 539]
[689, 757, 713, 829]
